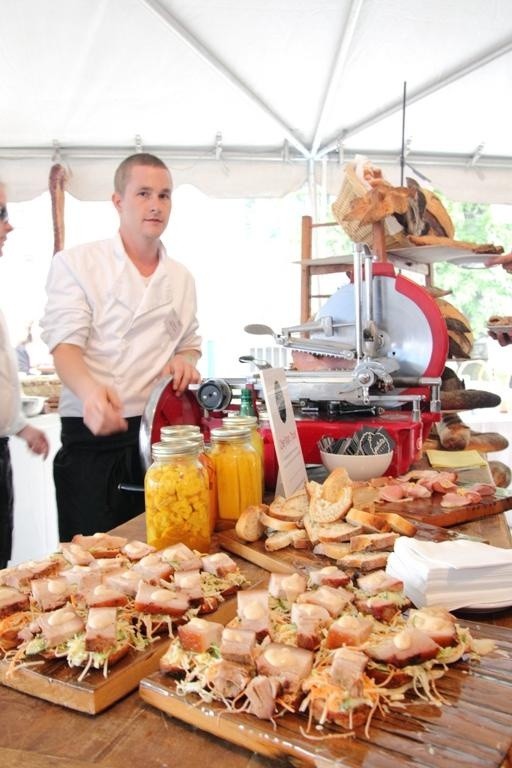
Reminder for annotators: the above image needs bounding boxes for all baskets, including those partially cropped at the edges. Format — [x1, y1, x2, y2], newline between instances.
[332, 162, 415, 251]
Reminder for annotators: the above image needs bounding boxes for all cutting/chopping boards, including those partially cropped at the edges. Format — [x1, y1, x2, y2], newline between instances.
[213, 503, 492, 582]
[0, 537, 244, 717]
[145, 608, 512, 768]
[362, 484, 512, 528]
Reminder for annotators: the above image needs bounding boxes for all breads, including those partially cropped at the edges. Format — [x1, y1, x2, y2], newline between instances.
[420, 187, 455, 238]
[409, 239, 504, 254]
[344, 165, 419, 225]
[239, 465, 417, 570]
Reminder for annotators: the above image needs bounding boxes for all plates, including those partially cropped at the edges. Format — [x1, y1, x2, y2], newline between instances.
[445, 255, 506, 269]
[486, 325, 512, 334]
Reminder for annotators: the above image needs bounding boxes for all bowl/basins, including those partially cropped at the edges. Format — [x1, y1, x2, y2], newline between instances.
[320, 450, 394, 481]
[21, 397, 49, 416]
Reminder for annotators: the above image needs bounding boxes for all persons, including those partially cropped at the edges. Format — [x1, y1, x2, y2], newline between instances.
[1, 181, 49, 569]
[479, 245, 512, 350]
[40, 153, 201, 542]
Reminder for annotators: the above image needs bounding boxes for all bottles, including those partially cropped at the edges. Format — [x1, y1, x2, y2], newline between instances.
[240, 389, 255, 417]
[245, 384, 259, 424]
[143, 481, 215, 556]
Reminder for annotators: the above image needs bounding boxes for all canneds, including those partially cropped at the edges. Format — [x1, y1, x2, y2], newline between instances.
[143, 415, 263, 549]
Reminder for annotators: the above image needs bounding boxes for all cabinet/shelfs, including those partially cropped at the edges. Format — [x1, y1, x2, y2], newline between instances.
[298, 216, 477, 341]
